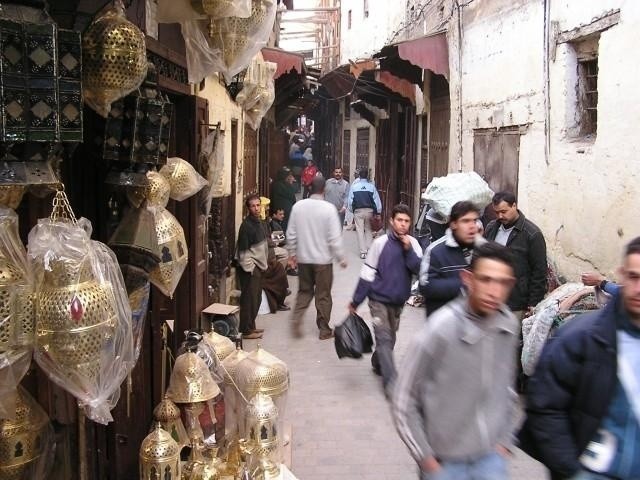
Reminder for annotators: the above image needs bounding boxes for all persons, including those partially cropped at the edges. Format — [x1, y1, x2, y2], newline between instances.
[349, 189, 546, 395]
[289, 126, 316, 193]
[385, 240, 526, 480]
[231, 160, 383, 340]
[526, 235, 640, 480]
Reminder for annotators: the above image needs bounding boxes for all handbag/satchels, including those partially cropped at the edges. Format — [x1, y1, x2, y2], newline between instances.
[421, 172, 495, 217]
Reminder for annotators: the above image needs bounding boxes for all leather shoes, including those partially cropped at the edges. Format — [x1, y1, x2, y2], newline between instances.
[279, 305, 290, 310]
[320, 330, 335, 339]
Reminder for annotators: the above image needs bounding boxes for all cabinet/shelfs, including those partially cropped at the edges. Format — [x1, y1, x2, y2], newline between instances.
[144, 94, 210, 413]
